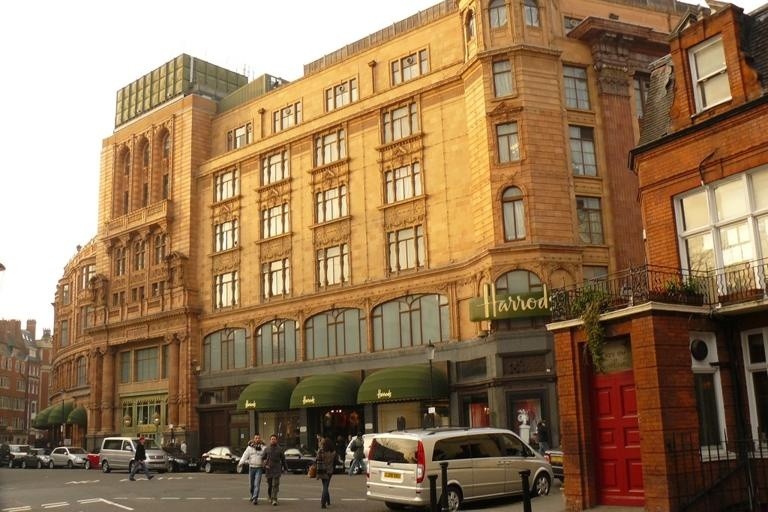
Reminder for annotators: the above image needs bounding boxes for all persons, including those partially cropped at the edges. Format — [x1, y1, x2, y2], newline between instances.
[336, 435, 346, 456]
[238, 432, 266, 505]
[261, 434, 290, 505]
[348, 431, 367, 476]
[129, 437, 154, 481]
[314, 438, 337, 509]
[316, 433, 324, 451]
[535, 422, 551, 452]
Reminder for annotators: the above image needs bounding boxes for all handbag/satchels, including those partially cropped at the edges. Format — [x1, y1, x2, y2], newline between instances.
[308, 464, 317, 479]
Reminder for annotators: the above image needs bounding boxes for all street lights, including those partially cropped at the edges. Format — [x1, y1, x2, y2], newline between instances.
[424, 339, 436, 427]
[59, 387, 67, 447]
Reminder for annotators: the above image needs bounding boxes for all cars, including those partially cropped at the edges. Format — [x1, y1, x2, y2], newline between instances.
[345, 434, 381, 476]
[283, 448, 318, 473]
[162, 446, 248, 473]
[544, 444, 564, 484]
[0, 444, 100, 469]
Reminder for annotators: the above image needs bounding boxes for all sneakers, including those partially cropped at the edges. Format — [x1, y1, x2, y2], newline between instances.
[129, 478, 136, 481]
[148, 475, 154, 480]
[268, 497, 278, 505]
[249, 496, 257, 504]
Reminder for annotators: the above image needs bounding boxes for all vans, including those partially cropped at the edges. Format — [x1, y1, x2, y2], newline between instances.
[366, 427, 553, 512]
[98, 436, 168, 473]
